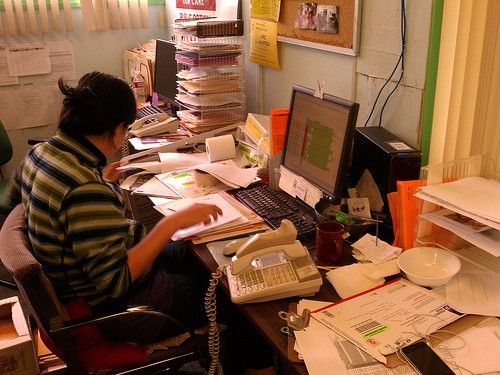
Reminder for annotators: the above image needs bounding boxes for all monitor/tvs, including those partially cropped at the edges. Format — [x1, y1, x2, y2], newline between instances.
[154, 39, 179, 117]
[280, 84, 360, 203]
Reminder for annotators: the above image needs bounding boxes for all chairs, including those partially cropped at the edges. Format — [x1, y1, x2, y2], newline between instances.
[0, 199, 221, 375]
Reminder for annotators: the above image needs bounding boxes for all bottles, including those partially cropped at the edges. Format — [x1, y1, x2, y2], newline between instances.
[256, 132, 270, 185]
[133, 71, 145, 107]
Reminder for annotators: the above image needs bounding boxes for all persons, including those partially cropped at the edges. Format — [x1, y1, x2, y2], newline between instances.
[0, 70, 279, 375]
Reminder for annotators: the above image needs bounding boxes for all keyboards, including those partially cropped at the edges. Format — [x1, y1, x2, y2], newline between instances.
[227, 180, 319, 239]
[136, 104, 165, 123]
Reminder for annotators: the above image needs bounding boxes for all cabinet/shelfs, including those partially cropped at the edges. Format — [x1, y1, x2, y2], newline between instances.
[173, 15, 243, 129]
[414, 153, 500, 283]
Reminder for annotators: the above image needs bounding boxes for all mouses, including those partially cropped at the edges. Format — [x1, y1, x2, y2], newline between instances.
[223, 237, 249, 255]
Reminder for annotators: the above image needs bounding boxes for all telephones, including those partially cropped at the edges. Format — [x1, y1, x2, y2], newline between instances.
[226, 239, 323, 304]
[130, 114, 180, 137]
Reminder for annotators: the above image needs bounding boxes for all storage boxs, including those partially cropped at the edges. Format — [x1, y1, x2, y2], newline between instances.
[0, 295, 41, 375]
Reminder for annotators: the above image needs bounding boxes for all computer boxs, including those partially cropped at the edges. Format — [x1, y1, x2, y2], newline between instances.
[353, 126, 422, 245]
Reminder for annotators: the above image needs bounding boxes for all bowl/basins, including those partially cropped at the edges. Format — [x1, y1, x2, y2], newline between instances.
[397, 247, 462, 287]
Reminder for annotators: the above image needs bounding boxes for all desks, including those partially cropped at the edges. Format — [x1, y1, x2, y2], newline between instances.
[185, 193, 396, 375]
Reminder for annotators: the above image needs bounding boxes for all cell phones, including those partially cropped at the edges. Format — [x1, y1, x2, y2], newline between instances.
[400, 341, 456, 375]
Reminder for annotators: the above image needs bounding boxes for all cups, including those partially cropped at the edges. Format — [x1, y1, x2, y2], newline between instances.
[315, 221, 345, 266]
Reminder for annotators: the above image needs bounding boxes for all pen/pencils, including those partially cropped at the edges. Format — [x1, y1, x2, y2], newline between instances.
[328, 212, 383, 223]
